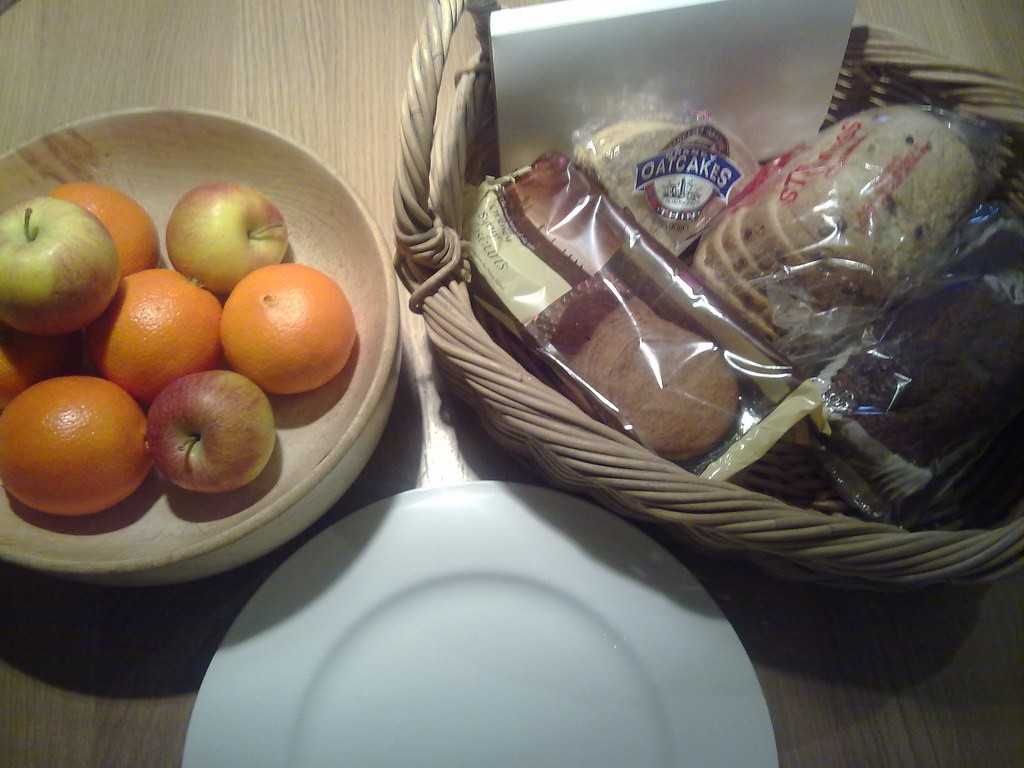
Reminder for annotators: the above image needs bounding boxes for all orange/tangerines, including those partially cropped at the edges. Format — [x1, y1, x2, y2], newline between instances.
[0, 181, 356, 517]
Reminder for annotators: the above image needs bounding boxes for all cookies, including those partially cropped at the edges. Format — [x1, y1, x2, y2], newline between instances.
[522, 263, 740, 461]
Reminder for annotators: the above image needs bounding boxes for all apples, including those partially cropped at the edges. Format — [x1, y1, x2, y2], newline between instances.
[166, 181, 290, 296]
[0, 196, 122, 337]
[146, 369, 277, 494]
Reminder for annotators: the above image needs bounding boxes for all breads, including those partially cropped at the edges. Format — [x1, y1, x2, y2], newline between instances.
[575, 95, 1024, 480]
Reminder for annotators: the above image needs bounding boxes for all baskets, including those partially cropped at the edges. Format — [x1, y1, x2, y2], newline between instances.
[393, 0, 1024, 585]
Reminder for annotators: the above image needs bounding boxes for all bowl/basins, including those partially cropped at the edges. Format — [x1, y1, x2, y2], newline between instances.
[1, 110, 402, 585]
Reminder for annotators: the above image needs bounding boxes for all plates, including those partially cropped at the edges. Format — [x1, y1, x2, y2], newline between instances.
[180, 479, 781, 768]
